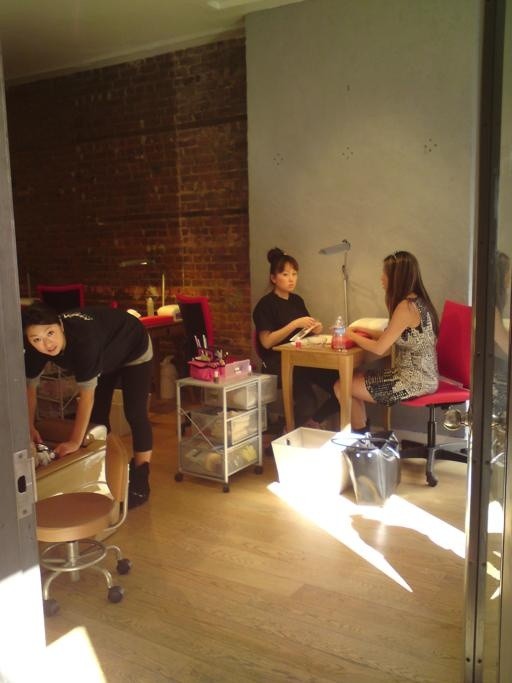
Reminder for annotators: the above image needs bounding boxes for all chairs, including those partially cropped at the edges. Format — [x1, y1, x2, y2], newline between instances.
[399, 299, 474, 487]
[33, 430, 137, 614]
[177, 296, 258, 374]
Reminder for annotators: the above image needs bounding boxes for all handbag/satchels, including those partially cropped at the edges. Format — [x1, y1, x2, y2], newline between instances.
[343, 430, 404, 506]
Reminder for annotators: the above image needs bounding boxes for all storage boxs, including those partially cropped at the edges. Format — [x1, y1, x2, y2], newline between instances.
[182, 373, 279, 477]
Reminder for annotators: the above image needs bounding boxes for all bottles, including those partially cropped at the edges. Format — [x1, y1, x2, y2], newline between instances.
[146, 295, 154, 315]
[332, 314, 347, 353]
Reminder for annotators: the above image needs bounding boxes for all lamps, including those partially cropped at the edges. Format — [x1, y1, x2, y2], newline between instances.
[319, 238, 355, 330]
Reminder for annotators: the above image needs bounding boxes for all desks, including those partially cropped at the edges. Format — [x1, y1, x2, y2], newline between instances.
[272, 335, 396, 433]
[125, 316, 183, 413]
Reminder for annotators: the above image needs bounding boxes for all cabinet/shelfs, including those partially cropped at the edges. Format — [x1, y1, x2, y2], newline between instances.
[170, 371, 263, 493]
[32, 361, 80, 423]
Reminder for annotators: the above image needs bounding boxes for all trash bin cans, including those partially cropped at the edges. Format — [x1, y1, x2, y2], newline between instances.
[159, 354, 181, 399]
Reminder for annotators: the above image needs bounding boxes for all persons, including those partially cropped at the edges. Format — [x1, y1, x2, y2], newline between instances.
[252, 244, 341, 437]
[20, 299, 156, 511]
[331, 248, 441, 434]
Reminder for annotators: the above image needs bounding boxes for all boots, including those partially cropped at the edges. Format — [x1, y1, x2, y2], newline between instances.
[120, 459, 150, 513]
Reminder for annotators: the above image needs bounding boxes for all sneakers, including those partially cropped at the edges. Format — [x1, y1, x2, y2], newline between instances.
[305, 417, 329, 430]
